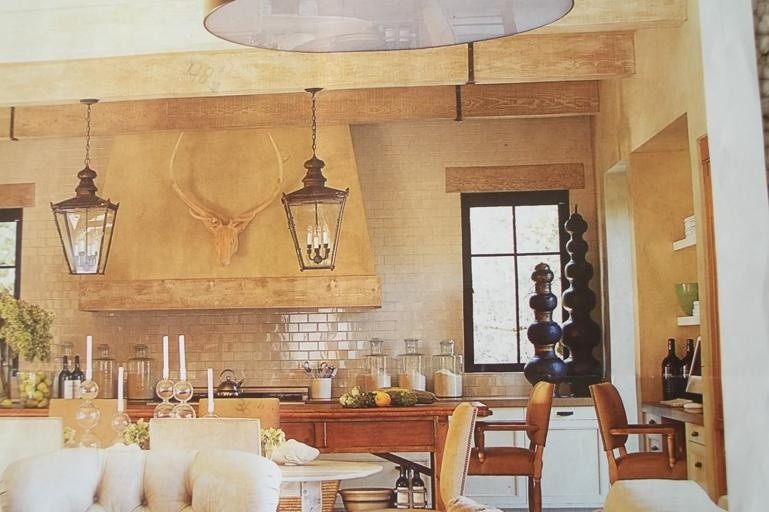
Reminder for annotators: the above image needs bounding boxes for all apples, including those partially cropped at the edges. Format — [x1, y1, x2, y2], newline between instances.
[1, 399, 12, 409]
[19, 371, 53, 407]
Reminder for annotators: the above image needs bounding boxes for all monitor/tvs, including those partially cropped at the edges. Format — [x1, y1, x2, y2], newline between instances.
[685, 336, 703, 394]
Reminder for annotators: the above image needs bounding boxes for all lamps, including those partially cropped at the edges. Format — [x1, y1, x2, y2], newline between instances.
[280, 88, 352, 270]
[48, 98, 118, 276]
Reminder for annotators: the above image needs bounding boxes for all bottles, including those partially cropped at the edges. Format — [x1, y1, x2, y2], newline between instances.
[364, 339, 463, 396]
[51, 342, 157, 399]
[395, 467, 426, 509]
[661, 337, 694, 401]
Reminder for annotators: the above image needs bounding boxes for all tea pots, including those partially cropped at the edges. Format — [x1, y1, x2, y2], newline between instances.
[216, 368, 245, 395]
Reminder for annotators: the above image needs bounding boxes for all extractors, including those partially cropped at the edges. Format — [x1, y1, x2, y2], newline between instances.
[79, 124, 381, 312]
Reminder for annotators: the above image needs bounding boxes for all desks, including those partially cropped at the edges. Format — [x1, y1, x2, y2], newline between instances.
[1, 400, 493, 512]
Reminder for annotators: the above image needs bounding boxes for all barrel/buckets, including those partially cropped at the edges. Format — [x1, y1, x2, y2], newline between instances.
[15, 371, 55, 409]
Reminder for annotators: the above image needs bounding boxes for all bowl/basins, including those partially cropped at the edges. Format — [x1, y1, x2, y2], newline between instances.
[675, 282, 699, 316]
[336, 487, 394, 512]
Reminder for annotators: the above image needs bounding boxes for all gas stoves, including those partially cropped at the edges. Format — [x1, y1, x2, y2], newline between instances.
[152, 388, 303, 404]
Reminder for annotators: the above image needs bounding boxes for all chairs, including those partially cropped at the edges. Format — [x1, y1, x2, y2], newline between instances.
[47, 399, 129, 444]
[199, 399, 280, 432]
[149, 418, 262, 458]
[590, 383, 686, 484]
[440, 402, 507, 512]
[1, 446, 279, 512]
[463, 380, 553, 512]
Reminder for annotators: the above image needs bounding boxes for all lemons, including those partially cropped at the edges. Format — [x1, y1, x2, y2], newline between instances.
[375, 392, 391, 406]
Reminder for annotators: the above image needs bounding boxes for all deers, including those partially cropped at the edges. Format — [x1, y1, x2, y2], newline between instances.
[167, 131, 290, 268]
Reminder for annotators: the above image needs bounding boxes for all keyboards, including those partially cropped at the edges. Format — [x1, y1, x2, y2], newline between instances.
[660, 398, 694, 407]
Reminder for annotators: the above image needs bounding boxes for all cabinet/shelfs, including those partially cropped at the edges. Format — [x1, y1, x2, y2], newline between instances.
[641, 403, 662, 453]
[684, 413, 708, 490]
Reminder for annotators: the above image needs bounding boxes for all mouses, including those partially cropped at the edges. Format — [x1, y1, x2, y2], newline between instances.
[683, 409, 703, 414]
[683, 402, 702, 408]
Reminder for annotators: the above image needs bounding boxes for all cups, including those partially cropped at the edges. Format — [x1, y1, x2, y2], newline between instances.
[310, 378, 331, 401]
